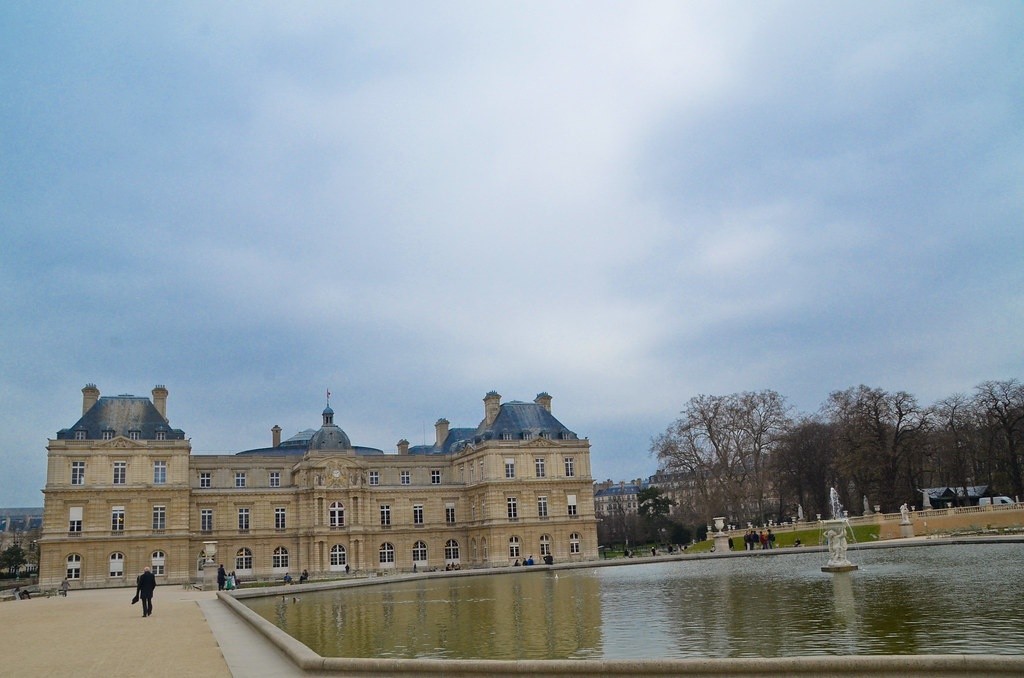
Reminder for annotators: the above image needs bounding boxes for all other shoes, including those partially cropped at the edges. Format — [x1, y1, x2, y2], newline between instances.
[141, 614, 146, 617]
[147, 612, 152, 616]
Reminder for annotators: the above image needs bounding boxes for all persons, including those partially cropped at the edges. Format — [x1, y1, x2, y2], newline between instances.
[900, 503, 910, 523]
[523, 557, 527, 565]
[60, 577, 71, 597]
[624, 529, 801, 559]
[299, 569, 308, 584]
[136, 567, 156, 617]
[413, 563, 416, 572]
[824, 525, 848, 563]
[446, 562, 460, 571]
[922, 490, 931, 506]
[863, 495, 869, 510]
[284, 572, 293, 585]
[13, 587, 21, 600]
[527, 553, 554, 565]
[515, 560, 521, 566]
[797, 504, 803, 520]
[217, 564, 227, 591]
[345, 564, 350, 573]
[229, 571, 241, 586]
[19, 590, 31, 600]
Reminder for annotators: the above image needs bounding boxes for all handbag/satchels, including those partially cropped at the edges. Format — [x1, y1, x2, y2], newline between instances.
[131, 595, 139, 604]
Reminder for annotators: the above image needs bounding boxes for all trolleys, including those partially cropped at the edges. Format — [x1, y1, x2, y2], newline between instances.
[225, 575, 236, 591]
